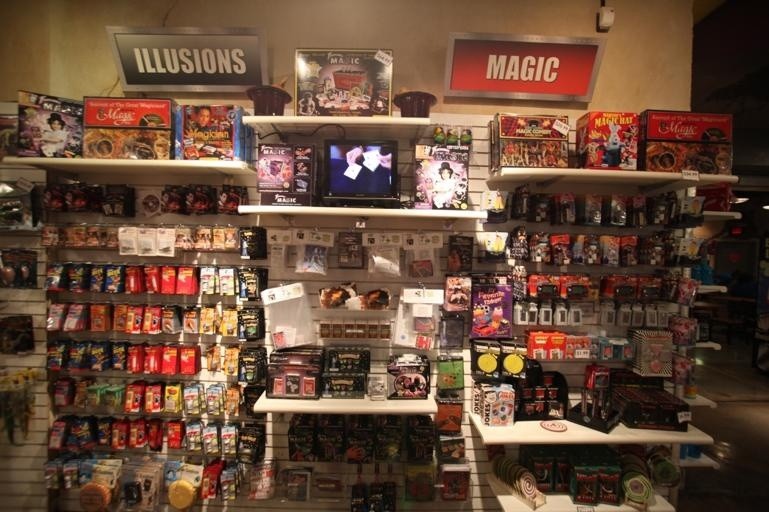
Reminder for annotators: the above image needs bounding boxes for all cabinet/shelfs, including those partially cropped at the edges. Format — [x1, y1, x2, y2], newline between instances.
[240, 114, 490, 417]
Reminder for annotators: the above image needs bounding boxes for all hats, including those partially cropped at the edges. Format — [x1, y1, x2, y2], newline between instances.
[437, 163, 452, 173]
[47, 113, 65, 125]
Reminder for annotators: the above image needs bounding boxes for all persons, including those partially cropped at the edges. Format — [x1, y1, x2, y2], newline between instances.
[346, 442, 366, 461]
[32, 112, 70, 160]
[188, 106, 222, 138]
[344, 142, 393, 171]
[41, 187, 54, 207]
[426, 162, 460, 209]
[158, 187, 240, 215]
[61, 190, 75, 209]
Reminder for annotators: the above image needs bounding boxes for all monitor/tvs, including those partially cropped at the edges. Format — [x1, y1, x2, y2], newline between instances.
[323, 139, 398, 207]
